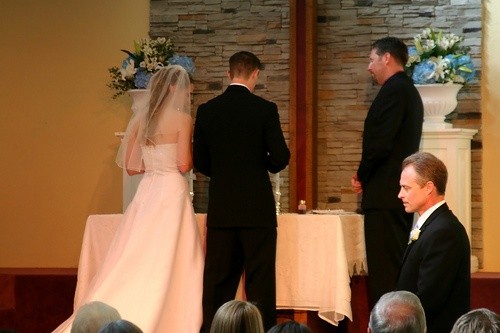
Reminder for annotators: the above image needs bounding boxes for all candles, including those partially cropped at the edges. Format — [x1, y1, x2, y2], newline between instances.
[275, 172, 281, 194]
[189, 169, 195, 195]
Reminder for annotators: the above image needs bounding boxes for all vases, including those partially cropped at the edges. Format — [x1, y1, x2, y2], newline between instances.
[411, 85, 463, 127]
[125, 88, 153, 114]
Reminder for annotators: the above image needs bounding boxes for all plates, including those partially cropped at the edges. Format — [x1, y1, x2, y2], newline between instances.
[312, 210, 343, 214]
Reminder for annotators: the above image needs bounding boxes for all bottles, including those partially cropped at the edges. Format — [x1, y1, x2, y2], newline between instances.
[298, 200, 307, 213]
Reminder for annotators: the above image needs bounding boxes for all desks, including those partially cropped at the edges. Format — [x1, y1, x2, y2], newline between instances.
[73, 211, 368, 327]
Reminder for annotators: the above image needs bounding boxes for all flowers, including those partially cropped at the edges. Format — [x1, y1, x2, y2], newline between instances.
[404, 27, 476, 85]
[105, 37, 196, 100]
[408, 227, 421, 245]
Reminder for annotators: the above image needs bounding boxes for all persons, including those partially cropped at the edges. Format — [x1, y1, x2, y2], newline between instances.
[210, 300, 265, 333]
[268, 320, 312, 333]
[451, 308, 500, 333]
[394, 151, 470, 333]
[352, 38, 424, 303]
[193, 51, 291, 333]
[99, 318, 143, 333]
[70, 302, 120, 333]
[53, 65, 204, 333]
[367, 291, 428, 333]
